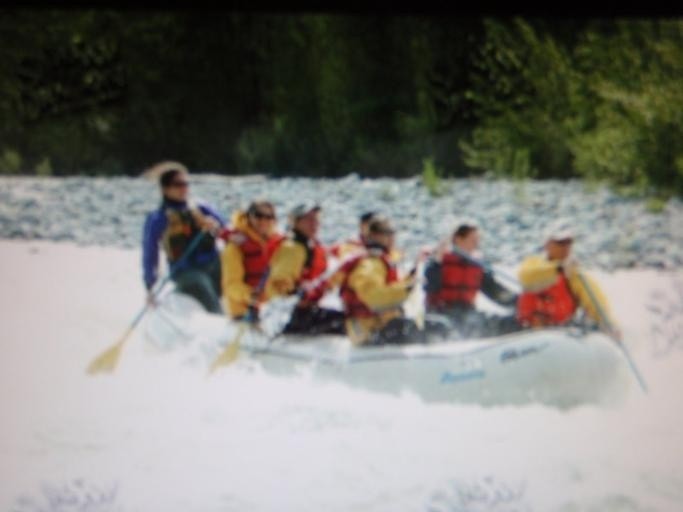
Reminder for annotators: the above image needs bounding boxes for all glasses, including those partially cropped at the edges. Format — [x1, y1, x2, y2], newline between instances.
[255, 212, 272, 220]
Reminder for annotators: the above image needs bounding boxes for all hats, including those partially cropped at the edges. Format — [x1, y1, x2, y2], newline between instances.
[291, 199, 321, 221]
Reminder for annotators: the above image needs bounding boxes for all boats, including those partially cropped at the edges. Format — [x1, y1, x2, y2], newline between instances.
[140, 259, 629, 409]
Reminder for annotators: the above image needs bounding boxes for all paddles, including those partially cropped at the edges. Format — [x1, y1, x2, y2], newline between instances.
[212, 265, 271, 367]
[88, 225, 207, 371]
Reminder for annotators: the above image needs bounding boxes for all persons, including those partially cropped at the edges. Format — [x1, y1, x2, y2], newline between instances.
[219, 195, 281, 321]
[337, 213, 455, 347]
[507, 220, 624, 344]
[140, 161, 224, 317]
[250, 202, 347, 338]
[324, 210, 402, 267]
[420, 218, 521, 338]
